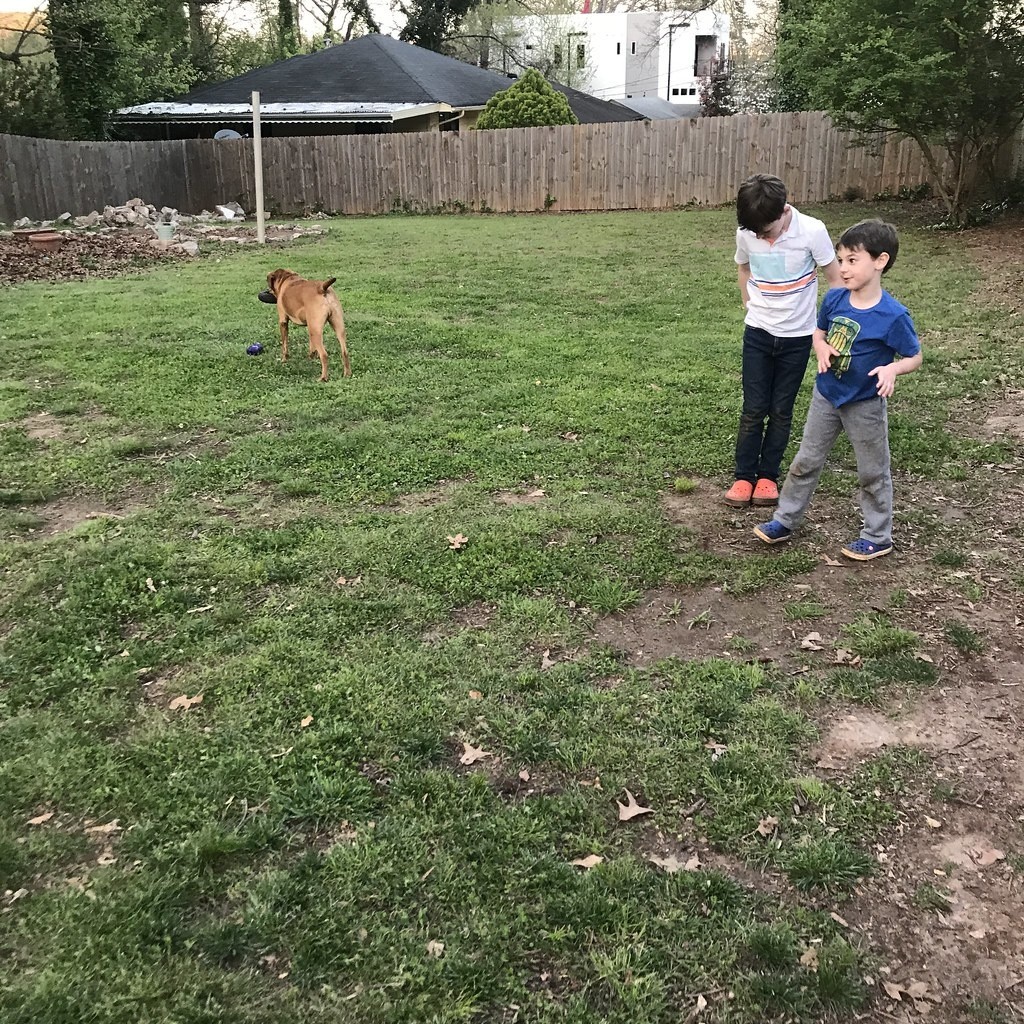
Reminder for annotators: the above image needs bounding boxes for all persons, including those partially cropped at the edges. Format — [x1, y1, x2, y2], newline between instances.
[753, 220, 922, 560]
[725, 173, 845, 507]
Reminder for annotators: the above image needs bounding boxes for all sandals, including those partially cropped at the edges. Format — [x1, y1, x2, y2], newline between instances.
[842, 537, 893, 561]
[754, 519, 792, 543]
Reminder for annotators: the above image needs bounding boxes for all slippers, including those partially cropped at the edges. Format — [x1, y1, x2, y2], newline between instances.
[723, 479, 754, 507]
[752, 478, 778, 506]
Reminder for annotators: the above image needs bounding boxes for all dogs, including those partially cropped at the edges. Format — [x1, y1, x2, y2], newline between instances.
[257, 266, 350, 385]
